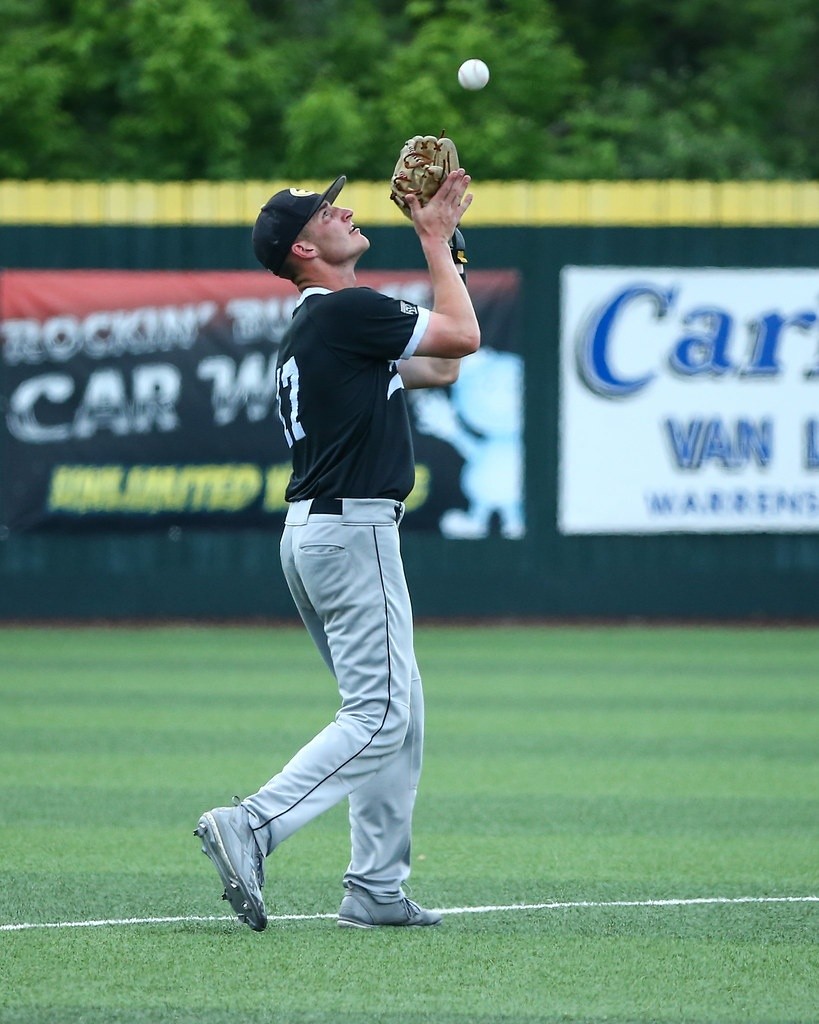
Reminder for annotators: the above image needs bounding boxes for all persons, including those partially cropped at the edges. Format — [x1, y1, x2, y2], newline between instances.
[193, 135, 480, 930]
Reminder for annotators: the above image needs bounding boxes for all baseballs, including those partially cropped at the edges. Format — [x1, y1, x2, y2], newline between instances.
[458, 59, 490, 91]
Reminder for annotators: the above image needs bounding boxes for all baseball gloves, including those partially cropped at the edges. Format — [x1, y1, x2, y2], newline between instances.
[391, 135, 460, 221]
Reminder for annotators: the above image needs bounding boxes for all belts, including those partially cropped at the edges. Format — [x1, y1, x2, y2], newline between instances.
[309, 498, 402, 523]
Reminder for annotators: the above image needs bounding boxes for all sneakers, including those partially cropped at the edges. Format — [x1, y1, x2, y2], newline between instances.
[337, 879, 442, 930]
[193, 795, 268, 932]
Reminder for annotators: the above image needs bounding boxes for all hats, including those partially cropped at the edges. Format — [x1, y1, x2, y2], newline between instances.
[252, 175, 347, 276]
